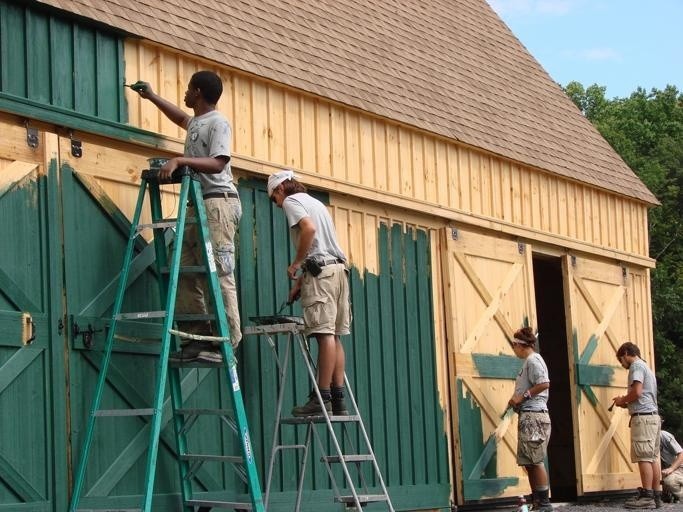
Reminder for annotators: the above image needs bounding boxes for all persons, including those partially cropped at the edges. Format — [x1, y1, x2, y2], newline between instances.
[508, 326, 553, 512]
[660, 429, 683, 503]
[267, 170, 352, 416]
[133, 70, 243, 363]
[612, 342, 664, 510]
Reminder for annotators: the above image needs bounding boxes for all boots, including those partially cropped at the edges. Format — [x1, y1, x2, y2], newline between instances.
[654, 490, 664, 508]
[291, 387, 333, 417]
[531, 489, 552, 512]
[625, 487, 657, 509]
[331, 387, 349, 416]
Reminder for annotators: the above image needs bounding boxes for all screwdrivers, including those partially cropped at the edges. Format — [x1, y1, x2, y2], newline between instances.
[277, 299, 295, 316]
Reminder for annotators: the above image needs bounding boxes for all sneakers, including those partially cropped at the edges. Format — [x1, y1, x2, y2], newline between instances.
[673, 497, 680, 504]
[197, 343, 235, 364]
[168, 340, 201, 362]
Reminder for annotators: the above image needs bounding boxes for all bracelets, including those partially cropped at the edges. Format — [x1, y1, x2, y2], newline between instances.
[526, 388, 532, 399]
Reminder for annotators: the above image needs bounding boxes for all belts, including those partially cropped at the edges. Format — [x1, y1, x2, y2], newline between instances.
[631, 411, 658, 417]
[518, 410, 548, 413]
[300, 258, 342, 270]
[187, 192, 238, 207]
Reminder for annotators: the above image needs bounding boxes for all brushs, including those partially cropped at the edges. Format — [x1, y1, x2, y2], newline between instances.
[500, 405, 511, 420]
[607, 401, 615, 411]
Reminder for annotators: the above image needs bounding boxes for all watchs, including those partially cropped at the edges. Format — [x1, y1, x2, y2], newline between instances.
[523, 391, 530, 401]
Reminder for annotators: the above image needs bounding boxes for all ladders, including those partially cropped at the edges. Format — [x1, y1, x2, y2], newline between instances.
[242, 322, 394, 511]
[68, 168, 264, 512]
